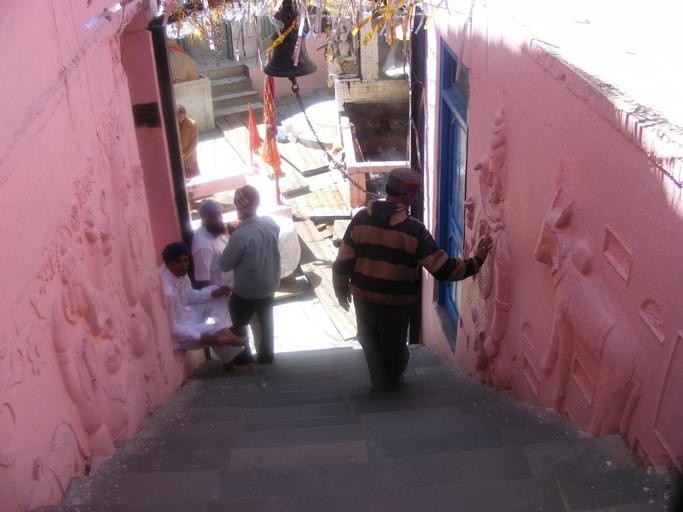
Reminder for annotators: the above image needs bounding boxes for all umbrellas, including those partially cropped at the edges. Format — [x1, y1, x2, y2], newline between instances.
[246, 101, 263, 161]
[260, 48, 287, 207]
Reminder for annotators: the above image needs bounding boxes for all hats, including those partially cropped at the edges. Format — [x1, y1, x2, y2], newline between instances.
[197, 198, 225, 218]
[232, 184, 261, 215]
[386, 166, 423, 194]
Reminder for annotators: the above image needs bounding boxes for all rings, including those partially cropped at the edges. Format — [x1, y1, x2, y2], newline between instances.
[481, 246, 487, 251]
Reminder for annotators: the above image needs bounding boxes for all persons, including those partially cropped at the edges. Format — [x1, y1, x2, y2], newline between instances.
[176, 103, 202, 178]
[217, 184, 282, 367]
[157, 241, 256, 377]
[331, 166, 493, 396]
[189, 198, 238, 298]
